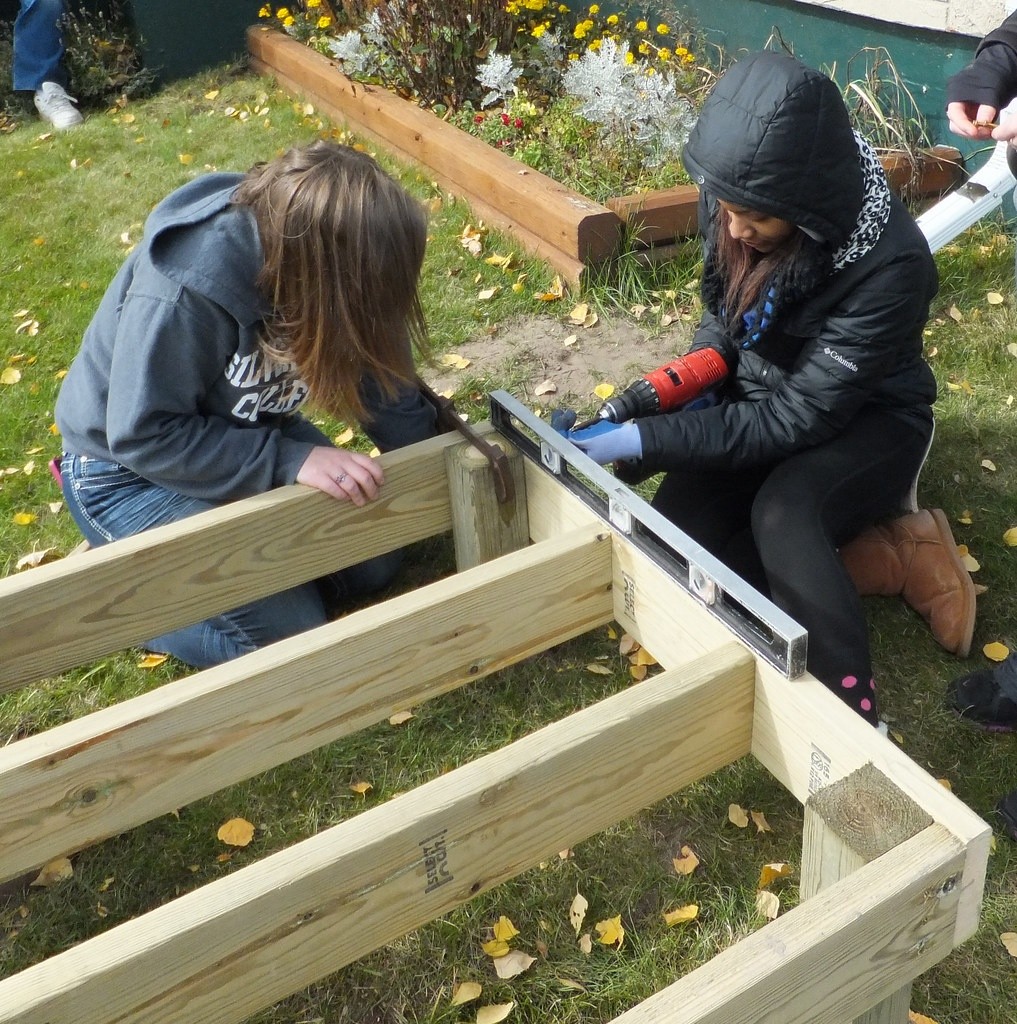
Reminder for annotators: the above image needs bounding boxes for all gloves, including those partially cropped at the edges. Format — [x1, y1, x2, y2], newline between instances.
[549, 408, 642, 467]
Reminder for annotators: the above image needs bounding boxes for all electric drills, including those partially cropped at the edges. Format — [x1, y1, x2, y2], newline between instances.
[566, 342, 739, 433]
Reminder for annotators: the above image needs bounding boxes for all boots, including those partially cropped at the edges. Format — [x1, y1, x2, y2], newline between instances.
[836, 509, 976, 659]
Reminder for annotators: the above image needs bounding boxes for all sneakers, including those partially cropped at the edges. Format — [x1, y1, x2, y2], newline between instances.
[34, 82, 84, 132]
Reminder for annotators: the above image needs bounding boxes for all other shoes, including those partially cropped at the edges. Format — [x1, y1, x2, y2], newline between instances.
[948, 669, 1017, 733]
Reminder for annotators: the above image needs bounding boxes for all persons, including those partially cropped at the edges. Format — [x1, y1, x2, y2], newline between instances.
[55, 139, 440, 668]
[946, 11, 1016, 730]
[12, 0, 87, 130]
[551, 50, 977, 736]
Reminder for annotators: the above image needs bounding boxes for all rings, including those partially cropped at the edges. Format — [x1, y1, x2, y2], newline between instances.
[334, 473, 349, 483]
